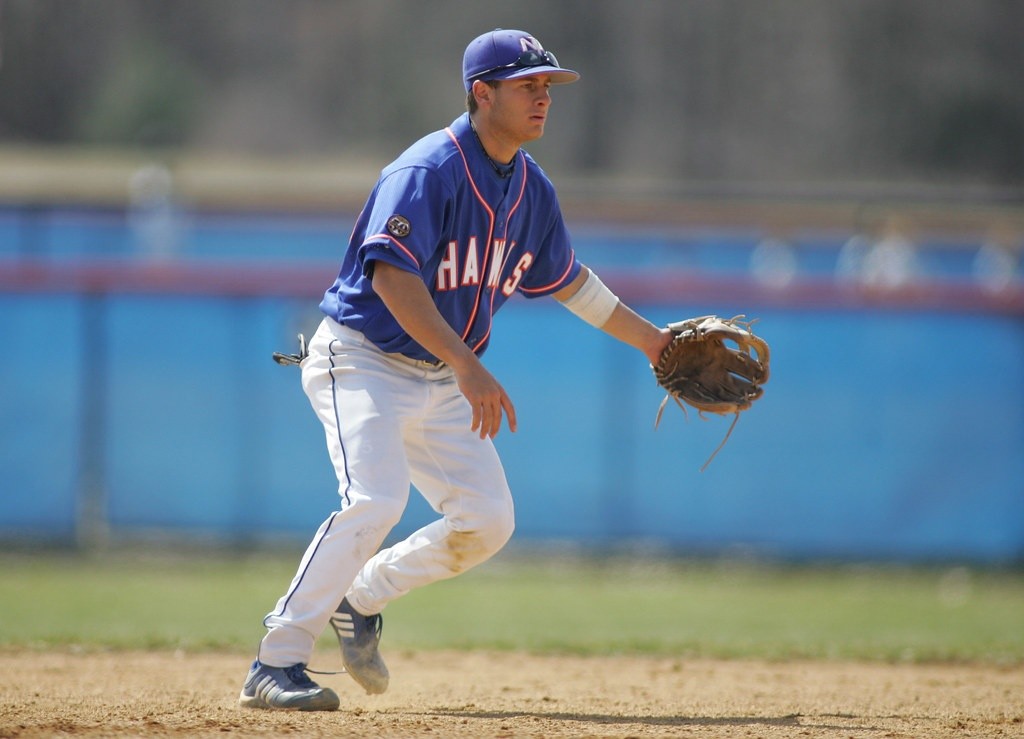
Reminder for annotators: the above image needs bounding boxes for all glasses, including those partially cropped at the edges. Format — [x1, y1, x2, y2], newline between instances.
[463, 50, 560, 80]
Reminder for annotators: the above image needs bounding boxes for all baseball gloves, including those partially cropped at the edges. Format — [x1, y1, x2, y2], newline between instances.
[647, 313, 770, 473]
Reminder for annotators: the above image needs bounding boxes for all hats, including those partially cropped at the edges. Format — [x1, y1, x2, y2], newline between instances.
[462, 29, 582, 91]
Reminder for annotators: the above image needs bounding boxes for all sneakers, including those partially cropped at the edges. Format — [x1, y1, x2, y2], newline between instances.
[328, 597, 390, 694]
[237, 661, 340, 711]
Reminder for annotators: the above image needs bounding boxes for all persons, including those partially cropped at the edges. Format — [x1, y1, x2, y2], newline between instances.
[241, 26, 769, 712]
